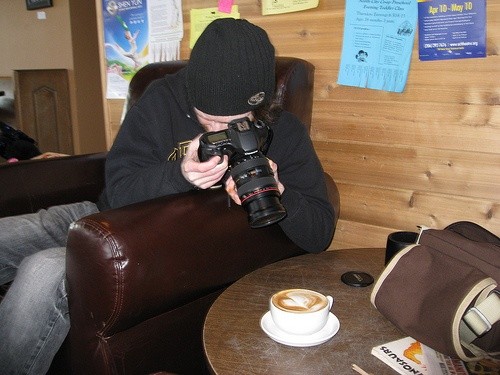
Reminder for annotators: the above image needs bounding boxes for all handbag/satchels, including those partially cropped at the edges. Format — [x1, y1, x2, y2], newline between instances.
[369, 221, 500, 361]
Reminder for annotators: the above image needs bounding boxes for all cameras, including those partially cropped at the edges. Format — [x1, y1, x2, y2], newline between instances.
[198, 117, 287, 228]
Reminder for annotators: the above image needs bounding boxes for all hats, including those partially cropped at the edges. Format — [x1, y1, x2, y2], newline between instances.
[187, 18, 276, 116]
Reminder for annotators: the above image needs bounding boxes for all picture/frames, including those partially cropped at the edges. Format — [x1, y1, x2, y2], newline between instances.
[25, 0, 53, 10]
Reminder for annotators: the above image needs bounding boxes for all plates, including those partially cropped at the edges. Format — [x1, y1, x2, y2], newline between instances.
[260, 310, 340, 348]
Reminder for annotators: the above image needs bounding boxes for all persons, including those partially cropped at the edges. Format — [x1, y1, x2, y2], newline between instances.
[0, 18, 336, 375]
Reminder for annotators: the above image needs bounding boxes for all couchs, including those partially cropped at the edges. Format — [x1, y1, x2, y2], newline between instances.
[0, 57, 341, 375]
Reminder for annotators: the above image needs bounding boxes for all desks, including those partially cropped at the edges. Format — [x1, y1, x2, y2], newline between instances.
[203, 248, 500, 375]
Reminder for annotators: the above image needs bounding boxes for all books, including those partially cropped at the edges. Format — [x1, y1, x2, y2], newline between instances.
[420, 342, 500, 375]
[371, 336, 430, 375]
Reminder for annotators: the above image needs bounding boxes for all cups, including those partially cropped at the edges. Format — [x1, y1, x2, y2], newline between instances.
[270, 288, 334, 334]
[385, 231, 418, 265]
